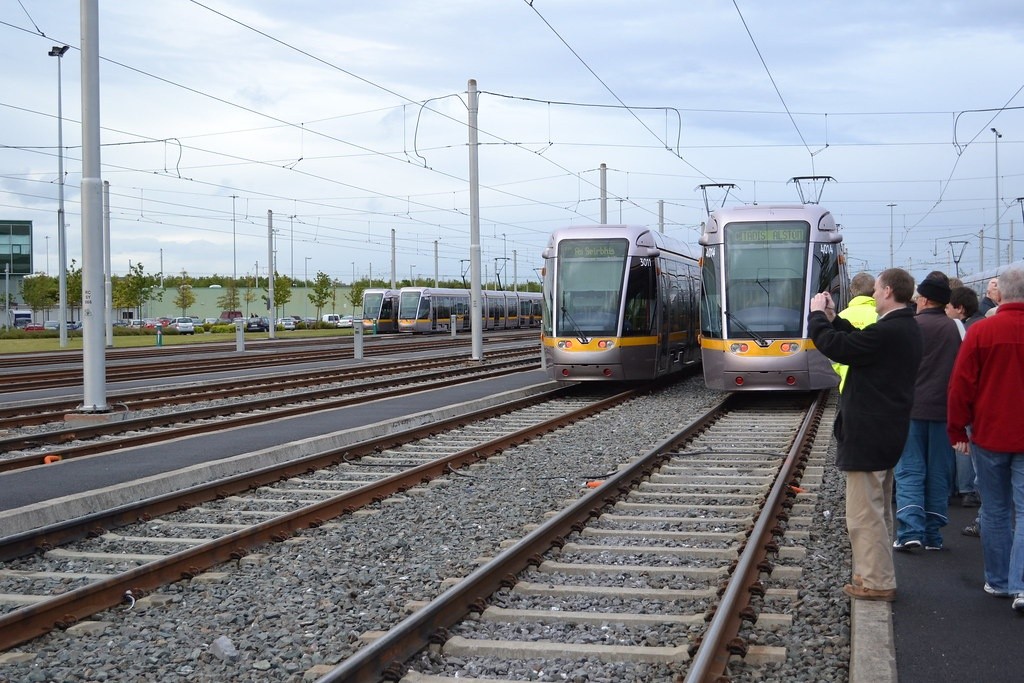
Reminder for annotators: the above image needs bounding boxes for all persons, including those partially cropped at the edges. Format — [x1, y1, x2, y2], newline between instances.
[805, 268, 1024, 612]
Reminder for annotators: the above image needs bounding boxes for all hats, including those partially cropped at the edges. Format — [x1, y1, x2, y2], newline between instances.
[917, 278, 951, 306]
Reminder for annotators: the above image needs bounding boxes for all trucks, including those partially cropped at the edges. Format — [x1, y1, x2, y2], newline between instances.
[9, 308, 33, 331]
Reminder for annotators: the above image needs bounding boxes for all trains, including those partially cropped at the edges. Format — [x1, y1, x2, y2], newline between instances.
[960, 263, 1015, 304]
[697, 202, 851, 391]
[362, 289, 402, 332]
[397, 287, 543, 335]
[539, 224, 701, 384]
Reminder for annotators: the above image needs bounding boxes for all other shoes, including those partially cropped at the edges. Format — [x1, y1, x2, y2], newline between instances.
[843, 583, 897, 601]
[1011, 593, 1024, 609]
[961, 518, 981, 537]
[852, 574, 863, 586]
[960, 493, 983, 509]
[892, 539, 922, 551]
[984, 582, 1014, 597]
[926, 544, 942, 550]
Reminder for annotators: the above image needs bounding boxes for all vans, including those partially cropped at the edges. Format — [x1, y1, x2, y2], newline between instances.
[321, 314, 340, 327]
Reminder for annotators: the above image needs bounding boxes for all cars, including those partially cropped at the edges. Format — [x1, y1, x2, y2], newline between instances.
[44, 320, 59, 330]
[202, 318, 216, 325]
[113, 317, 172, 328]
[247, 317, 276, 333]
[304, 316, 317, 327]
[286, 315, 303, 324]
[66, 320, 82, 330]
[23, 322, 45, 331]
[217, 316, 250, 325]
[337, 315, 355, 328]
[192, 319, 204, 327]
[170, 317, 194, 335]
[276, 318, 296, 331]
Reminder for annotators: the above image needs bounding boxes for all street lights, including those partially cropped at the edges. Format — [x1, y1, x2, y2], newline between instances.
[49, 45, 71, 350]
[305, 256, 312, 329]
[886, 201, 899, 269]
[409, 264, 416, 287]
[989, 127, 1003, 269]
